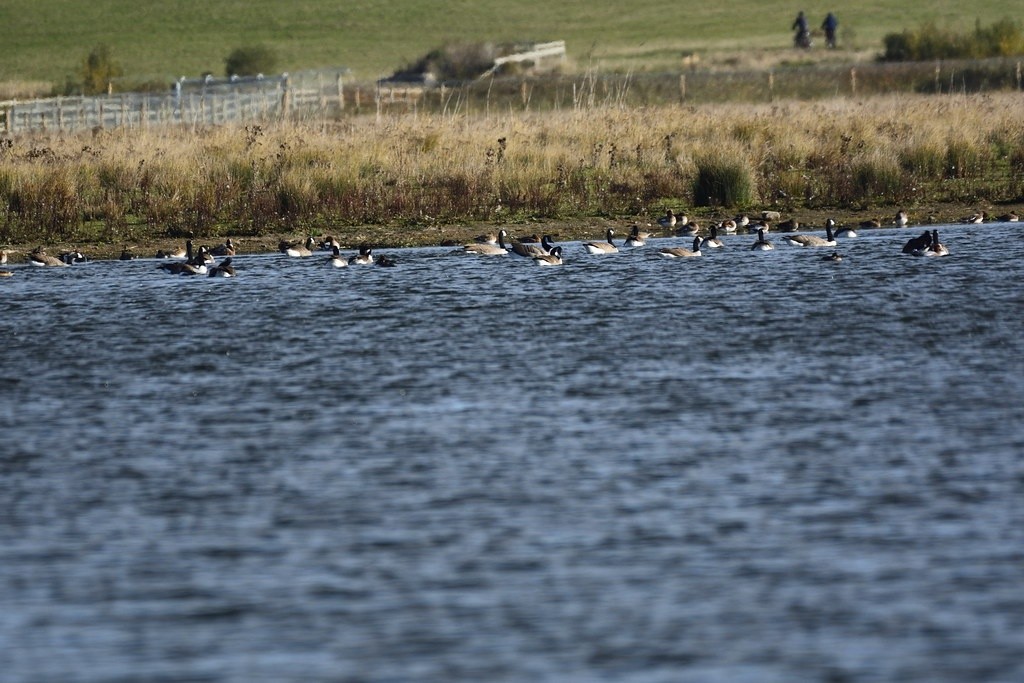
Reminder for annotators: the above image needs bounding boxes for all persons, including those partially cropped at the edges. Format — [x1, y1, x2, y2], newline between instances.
[792, 9, 838, 49]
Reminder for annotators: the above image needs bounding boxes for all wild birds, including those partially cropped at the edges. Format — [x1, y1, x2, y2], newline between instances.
[623, 225, 647, 247]
[996, 210, 1019, 222]
[818, 252, 842, 262]
[582, 224, 620, 255]
[20, 245, 97, 268]
[901, 229, 950, 257]
[717, 213, 775, 251]
[895, 209, 908, 226]
[656, 209, 699, 237]
[460, 229, 563, 267]
[0, 247, 20, 264]
[958, 211, 986, 224]
[776, 217, 799, 232]
[155, 238, 236, 279]
[324, 245, 398, 270]
[654, 225, 725, 258]
[780, 218, 858, 246]
[277, 235, 341, 258]
[859, 217, 881, 229]
